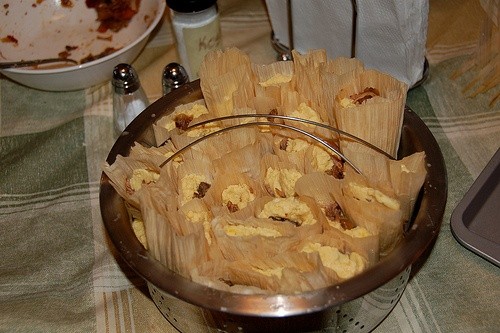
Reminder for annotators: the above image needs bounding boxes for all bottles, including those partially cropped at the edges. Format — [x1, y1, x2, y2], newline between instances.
[112, 63, 150, 135]
[166, 0, 223, 83]
[162, 62, 191, 96]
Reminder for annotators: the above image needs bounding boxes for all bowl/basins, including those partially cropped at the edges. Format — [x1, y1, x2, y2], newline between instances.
[0, 0, 166, 91]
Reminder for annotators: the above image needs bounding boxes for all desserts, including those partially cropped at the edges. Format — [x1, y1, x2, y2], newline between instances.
[105, 46, 427, 294]
